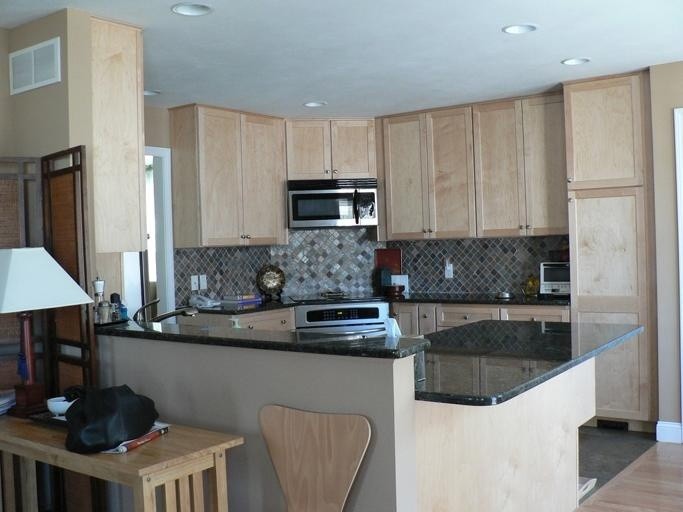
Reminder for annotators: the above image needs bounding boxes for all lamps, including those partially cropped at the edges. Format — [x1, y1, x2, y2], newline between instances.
[2, 239, 104, 419]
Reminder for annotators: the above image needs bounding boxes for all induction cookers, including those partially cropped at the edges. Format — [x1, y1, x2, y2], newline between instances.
[289, 291, 386, 304]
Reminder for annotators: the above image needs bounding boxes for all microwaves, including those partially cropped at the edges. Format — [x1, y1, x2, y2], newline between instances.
[287, 178, 378, 228]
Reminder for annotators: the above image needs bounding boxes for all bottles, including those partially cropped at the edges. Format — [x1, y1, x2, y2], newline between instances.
[92, 277, 127, 324]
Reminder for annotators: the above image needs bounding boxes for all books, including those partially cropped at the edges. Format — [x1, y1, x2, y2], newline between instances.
[219, 294, 262, 305]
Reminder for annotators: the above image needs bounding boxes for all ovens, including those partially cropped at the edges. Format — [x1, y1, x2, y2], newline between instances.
[295, 302, 389, 335]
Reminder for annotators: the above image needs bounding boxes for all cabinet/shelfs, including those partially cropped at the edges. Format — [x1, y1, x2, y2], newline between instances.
[91, 15, 149, 257]
[390, 303, 438, 339]
[559, 68, 658, 437]
[469, 90, 570, 241]
[176, 307, 296, 333]
[371, 102, 475, 246]
[168, 102, 290, 253]
[281, 116, 374, 183]
[434, 296, 572, 336]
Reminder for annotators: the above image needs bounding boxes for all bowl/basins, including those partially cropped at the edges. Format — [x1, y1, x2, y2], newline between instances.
[47, 397, 78, 414]
[496, 291, 515, 298]
[383, 285, 405, 297]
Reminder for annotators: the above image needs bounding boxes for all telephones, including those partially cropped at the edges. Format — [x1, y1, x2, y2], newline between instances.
[188, 294, 221, 306]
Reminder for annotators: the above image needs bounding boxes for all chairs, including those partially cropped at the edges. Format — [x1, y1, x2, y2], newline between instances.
[252, 398, 374, 512]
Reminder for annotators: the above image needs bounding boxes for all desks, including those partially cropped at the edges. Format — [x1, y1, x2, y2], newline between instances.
[1, 399, 244, 511]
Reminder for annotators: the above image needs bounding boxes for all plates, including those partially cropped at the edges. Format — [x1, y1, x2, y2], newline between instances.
[258, 265, 285, 294]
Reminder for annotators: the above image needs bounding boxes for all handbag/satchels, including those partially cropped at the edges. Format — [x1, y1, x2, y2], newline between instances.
[61, 382, 160, 455]
